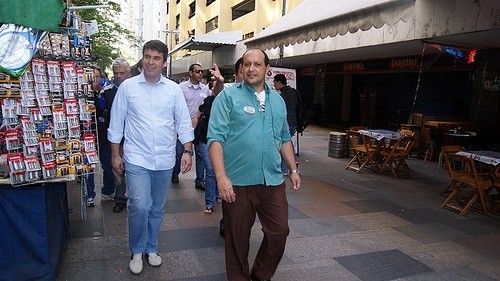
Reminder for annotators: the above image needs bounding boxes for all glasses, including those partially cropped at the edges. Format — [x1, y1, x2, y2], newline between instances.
[194, 70, 204, 74]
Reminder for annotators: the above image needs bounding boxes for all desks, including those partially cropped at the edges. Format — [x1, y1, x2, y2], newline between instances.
[425, 121, 478, 144]
[358, 129, 407, 149]
[456, 150, 500, 195]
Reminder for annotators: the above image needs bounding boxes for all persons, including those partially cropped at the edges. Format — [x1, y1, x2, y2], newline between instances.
[77, 57, 144, 213]
[207, 48, 301, 281]
[274, 73, 306, 175]
[173, 64, 214, 192]
[195, 95, 223, 214]
[104, 39, 194, 275]
[208, 56, 252, 96]
[97, 59, 132, 213]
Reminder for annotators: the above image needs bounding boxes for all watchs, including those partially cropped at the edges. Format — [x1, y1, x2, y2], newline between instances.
[183, 149, 193, 156]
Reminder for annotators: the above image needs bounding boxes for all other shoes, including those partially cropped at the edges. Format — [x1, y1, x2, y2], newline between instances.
[205, 207, 212, 215]
[113, 202, 126, 212]
[195, 182, 205, 191]
[171, 175, 179, 184]
[282, 170, 288, 175]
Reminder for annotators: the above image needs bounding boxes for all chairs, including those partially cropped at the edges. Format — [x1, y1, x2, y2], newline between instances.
[345, 127, 416, 178]
[439, 145, 500, 217]
[401, 113, 434, 162]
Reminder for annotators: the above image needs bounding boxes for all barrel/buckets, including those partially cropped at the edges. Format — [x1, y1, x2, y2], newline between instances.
[328, 132, 348, 158]
[348, 133, 364, 158]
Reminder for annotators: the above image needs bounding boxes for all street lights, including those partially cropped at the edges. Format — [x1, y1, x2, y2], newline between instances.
[162, 29, 180, 75]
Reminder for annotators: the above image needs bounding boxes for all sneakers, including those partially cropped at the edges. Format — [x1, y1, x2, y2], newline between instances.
[101, 192, 115, 201]
[128, 252, 144, 275]
[85, 197, 96, 207]
[145, 252, 162, 266]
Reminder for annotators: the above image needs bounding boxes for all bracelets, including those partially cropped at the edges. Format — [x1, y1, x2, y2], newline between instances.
[289, 169, 298, 174]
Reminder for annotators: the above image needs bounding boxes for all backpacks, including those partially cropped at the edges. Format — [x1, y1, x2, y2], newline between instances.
[293, 88, 308, 133]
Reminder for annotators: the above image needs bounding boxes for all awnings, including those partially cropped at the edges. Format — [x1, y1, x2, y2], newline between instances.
[167, 31, 238, 57]
